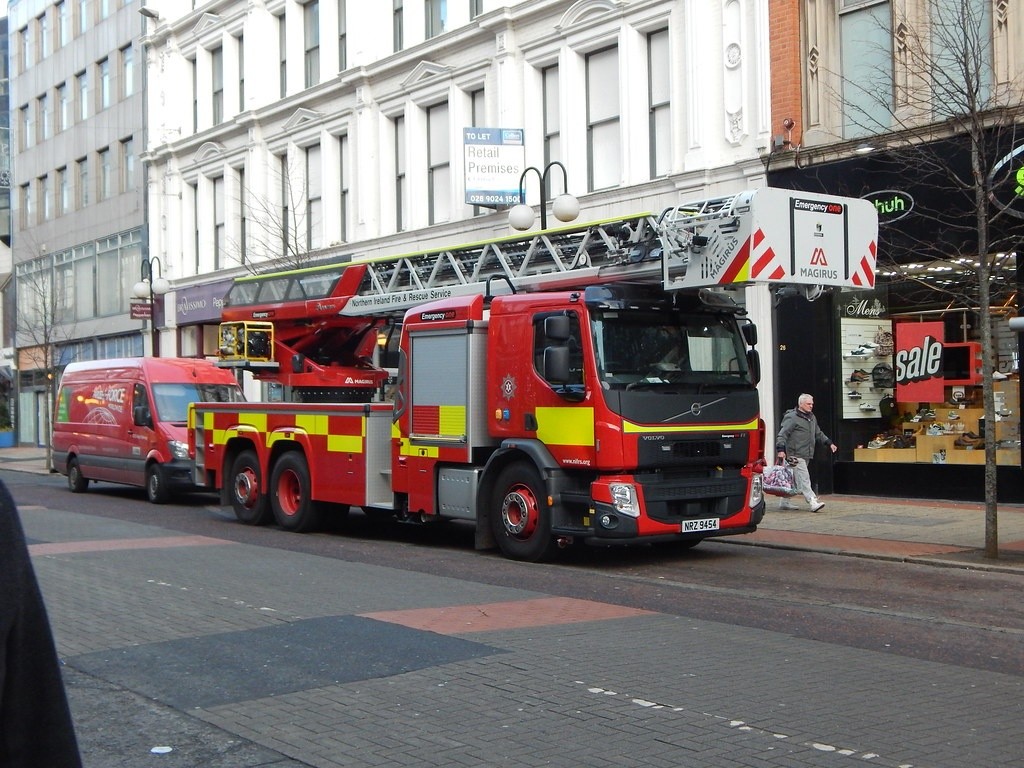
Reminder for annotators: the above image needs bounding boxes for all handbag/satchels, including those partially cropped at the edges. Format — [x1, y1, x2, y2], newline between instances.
[762, 455, 796, 496]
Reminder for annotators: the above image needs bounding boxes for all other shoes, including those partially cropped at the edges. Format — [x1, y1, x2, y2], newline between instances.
[949, 397, 976, 405]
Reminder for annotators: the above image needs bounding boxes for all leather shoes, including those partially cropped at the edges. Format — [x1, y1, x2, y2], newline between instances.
[851, 368, 872, 382]
[954, 431, 982, 446]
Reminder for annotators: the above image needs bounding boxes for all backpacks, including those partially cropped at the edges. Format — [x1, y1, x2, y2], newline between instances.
[872, 362, 895, 388]
[874, 326, 894, 356]
[879, 394, 899, 418]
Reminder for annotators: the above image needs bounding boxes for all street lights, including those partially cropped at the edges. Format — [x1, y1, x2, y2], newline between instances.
[508, 159, 580, 233]
[134, 257, 170, 359]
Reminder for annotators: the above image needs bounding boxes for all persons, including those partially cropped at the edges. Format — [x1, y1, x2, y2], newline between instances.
[776, 393, 838, 512]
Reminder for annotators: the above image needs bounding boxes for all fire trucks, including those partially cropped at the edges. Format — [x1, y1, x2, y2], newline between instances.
[187, 188, 881, 565]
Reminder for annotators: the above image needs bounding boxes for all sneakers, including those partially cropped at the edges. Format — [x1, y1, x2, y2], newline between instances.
[904, 408, 965, 436]
[867, 433, 893, 449]
[779, 498, 799, 510]
[859, 402, 874, 409]
[850, 342, 879, 355]
[848, 390, 862, 396]
[807, 496, 825, 512]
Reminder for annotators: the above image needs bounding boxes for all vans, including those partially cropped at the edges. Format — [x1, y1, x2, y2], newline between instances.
[50, 357, 247, 504]
